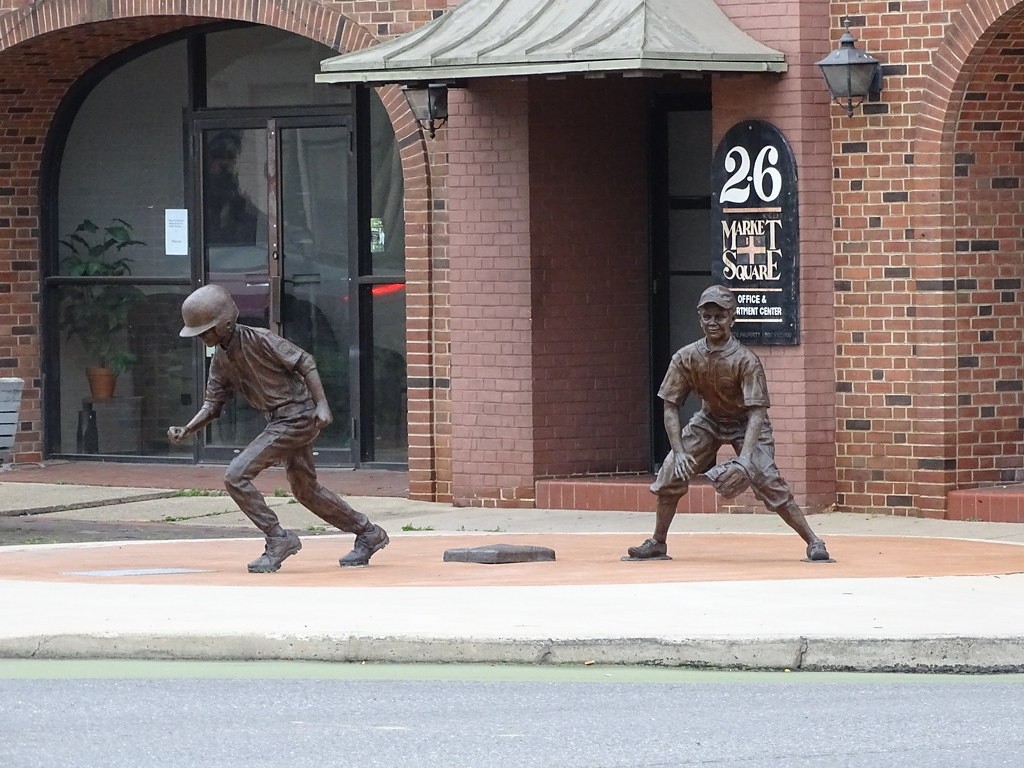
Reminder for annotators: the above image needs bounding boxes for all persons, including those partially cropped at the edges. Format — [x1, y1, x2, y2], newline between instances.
[627, 285, 830, 560]
[167, 284, 390, 574]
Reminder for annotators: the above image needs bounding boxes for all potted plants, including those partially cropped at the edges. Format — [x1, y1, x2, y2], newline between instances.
[58, 217, 144, 398]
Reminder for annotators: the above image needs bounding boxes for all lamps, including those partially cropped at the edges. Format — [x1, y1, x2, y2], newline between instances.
[814, 15, 883, 118]
[400, 80, 448, 139]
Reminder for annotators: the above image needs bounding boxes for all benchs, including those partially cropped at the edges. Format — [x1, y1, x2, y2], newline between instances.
[0, 378, 24, 465]
